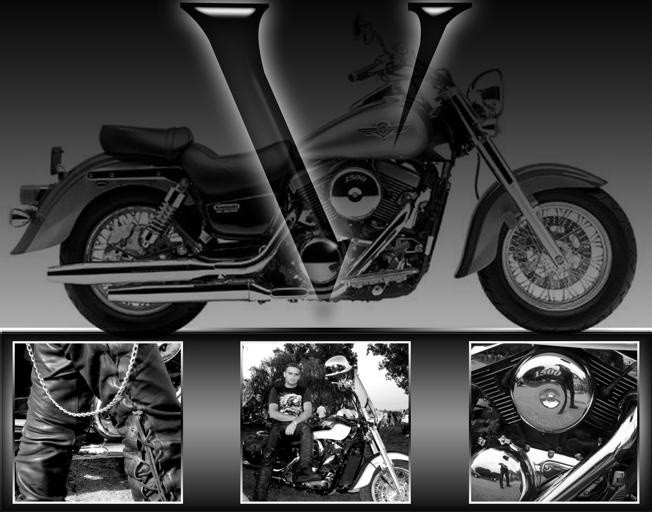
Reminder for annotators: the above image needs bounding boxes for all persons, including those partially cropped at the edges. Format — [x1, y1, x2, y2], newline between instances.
[554, 363, 578, 413]
[14, 342, 183, 503]
[252, 363, 321, 501]
[495, 454, 516, 489]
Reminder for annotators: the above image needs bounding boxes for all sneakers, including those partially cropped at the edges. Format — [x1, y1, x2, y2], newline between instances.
[253, 490, 268, 501]
[295, 466, 321, 483]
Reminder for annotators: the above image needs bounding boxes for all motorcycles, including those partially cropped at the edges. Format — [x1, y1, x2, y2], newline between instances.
[243, 355, 410, 503]
[8, 46, 638, 336]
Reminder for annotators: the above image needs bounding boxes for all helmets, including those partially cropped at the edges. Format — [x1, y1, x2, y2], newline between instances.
[324, 355, 353, 377]
